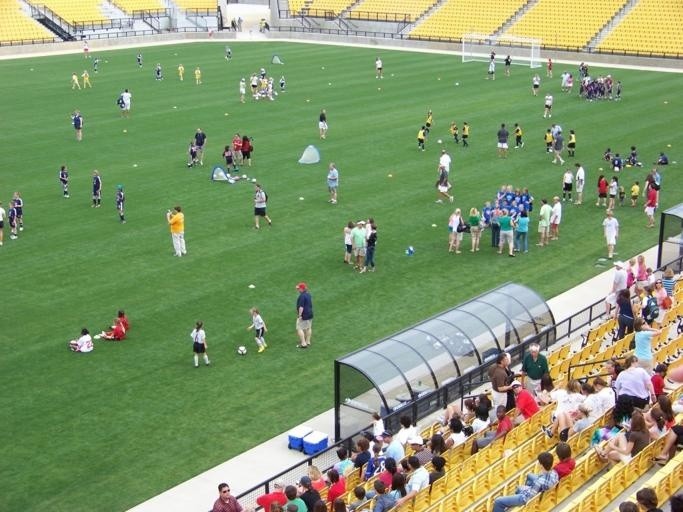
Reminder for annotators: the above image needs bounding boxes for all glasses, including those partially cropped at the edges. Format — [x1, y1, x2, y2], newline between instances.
[512, 384, 520, 389]
[221, 489, 230, 493]
[656, 281, 663, 284]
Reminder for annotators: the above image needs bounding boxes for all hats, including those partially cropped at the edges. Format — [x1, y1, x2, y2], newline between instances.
[295, 284, 306, 290]
[359, 430, 375, 440]
[274, 479, 288, 488]
[511, 380, 520, 386]
[408, 436, 423, 445]
[380, 430, 392, 438]
[297, 477, 310, 484]
[357, 220, 366, 226]
[613, 261, 623, 268]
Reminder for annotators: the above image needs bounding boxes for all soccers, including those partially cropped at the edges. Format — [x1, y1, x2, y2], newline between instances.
[237, 346, 246, 354]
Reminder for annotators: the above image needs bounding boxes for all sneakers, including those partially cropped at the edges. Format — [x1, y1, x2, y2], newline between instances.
[600, 315, 612, 319]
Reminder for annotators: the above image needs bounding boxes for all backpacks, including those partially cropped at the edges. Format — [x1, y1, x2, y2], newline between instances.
[646, 298, 659, 319]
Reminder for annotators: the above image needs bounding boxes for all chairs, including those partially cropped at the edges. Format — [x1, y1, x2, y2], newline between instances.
[1, 1, 218, 47]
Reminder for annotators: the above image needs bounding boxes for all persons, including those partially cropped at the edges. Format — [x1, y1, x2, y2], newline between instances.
[189, 282, 314, 367]
[0, 192, 23, 245]
[70, 308, 129, 352]
[417, 46, 669, 259]
[375, 56, 385, 82]
[344, 219, 376, 274]
[231, 17, 270, 33]
[60, 42, 338, 259]
[212, 256, 683, 512]
